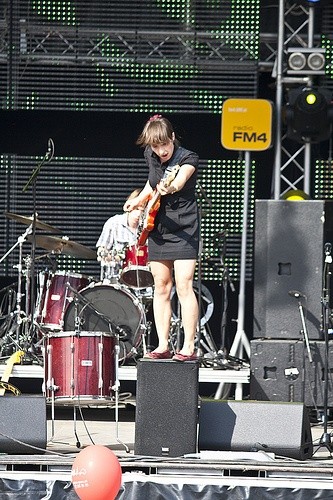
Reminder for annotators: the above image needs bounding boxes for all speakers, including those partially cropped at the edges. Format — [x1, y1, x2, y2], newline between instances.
[0, 394, 47, 454]
[254, 198, 327, 341]
[134, 359, 314, 460]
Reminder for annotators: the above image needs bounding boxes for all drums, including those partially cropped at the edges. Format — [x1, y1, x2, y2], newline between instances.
[120, 242, 154, 290]
[62, 282, 146, 365]
[35, 269, 98, 333]
[42, 330, 118, 408]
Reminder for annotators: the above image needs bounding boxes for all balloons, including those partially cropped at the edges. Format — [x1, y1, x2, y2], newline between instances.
[71, 445, 122, 500]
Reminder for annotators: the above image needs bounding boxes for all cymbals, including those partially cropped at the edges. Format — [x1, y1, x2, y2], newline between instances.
[2, 210, 61, 233]
[26, 234, 98, 260]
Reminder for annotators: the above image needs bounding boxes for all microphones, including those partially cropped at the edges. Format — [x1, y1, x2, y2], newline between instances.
[199, 186, 210, 204]
[116, 326, 131, 340]
[288, 290, 306, 298]
[48, 140, 51, 155]
[324, 243, 333, 255]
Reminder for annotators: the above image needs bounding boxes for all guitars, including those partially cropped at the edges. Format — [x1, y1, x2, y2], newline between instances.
[136, 163, 180, 246]
[0, 350, 25, 398]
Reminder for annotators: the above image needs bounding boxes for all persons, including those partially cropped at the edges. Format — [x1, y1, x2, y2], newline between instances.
[96, 188, 150, 282]
[123, 114, 201, 360]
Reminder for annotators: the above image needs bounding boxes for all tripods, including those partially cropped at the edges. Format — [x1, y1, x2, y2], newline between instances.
[0, 220, 46, 366]
[313, 255, 333, 453]
[198, 194, 251, 368]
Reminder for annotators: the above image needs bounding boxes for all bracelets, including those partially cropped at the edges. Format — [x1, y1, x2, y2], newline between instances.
[172, 185, 178, 194]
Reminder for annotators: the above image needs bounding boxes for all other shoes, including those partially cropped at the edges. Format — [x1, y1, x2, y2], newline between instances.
[172, 351, 197, 361]
[143, 349, 170, 361]
